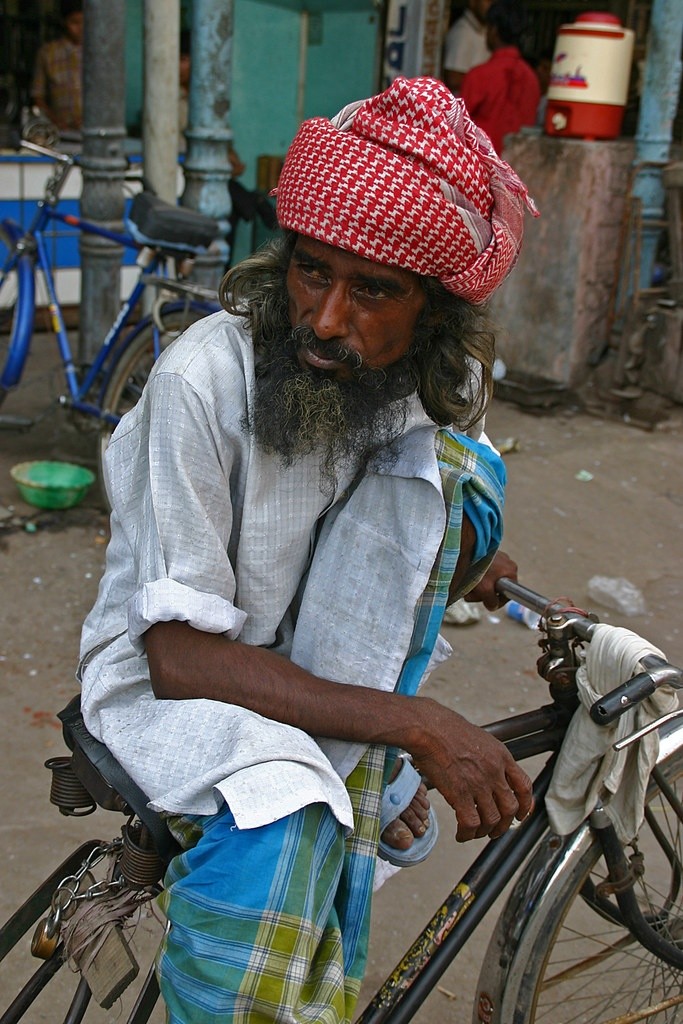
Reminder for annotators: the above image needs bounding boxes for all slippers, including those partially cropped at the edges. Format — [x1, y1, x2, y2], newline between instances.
[377, 756, 439, 867]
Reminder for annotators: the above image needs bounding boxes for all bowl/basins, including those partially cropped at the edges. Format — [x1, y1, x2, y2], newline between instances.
[10, 460, 96, 508]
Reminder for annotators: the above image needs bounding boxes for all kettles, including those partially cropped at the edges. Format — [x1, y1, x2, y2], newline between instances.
[538, 12, 636, 140]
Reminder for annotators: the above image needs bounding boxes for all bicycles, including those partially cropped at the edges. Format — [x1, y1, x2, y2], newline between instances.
[0, 126, 245, 519]
[0, 576, 681, 1024]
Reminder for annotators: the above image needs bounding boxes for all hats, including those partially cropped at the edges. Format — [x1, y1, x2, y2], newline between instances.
[269, 74, 541, 305]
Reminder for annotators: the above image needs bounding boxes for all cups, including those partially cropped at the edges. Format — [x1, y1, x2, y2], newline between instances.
[505, 598, 547, 630]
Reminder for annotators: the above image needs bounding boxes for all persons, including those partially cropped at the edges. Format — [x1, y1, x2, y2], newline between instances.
[75, 76, 532, 1024]
[179, 29, 245, 178]
[29, 2, 85, 132]
[443, 0, 552, 157]
